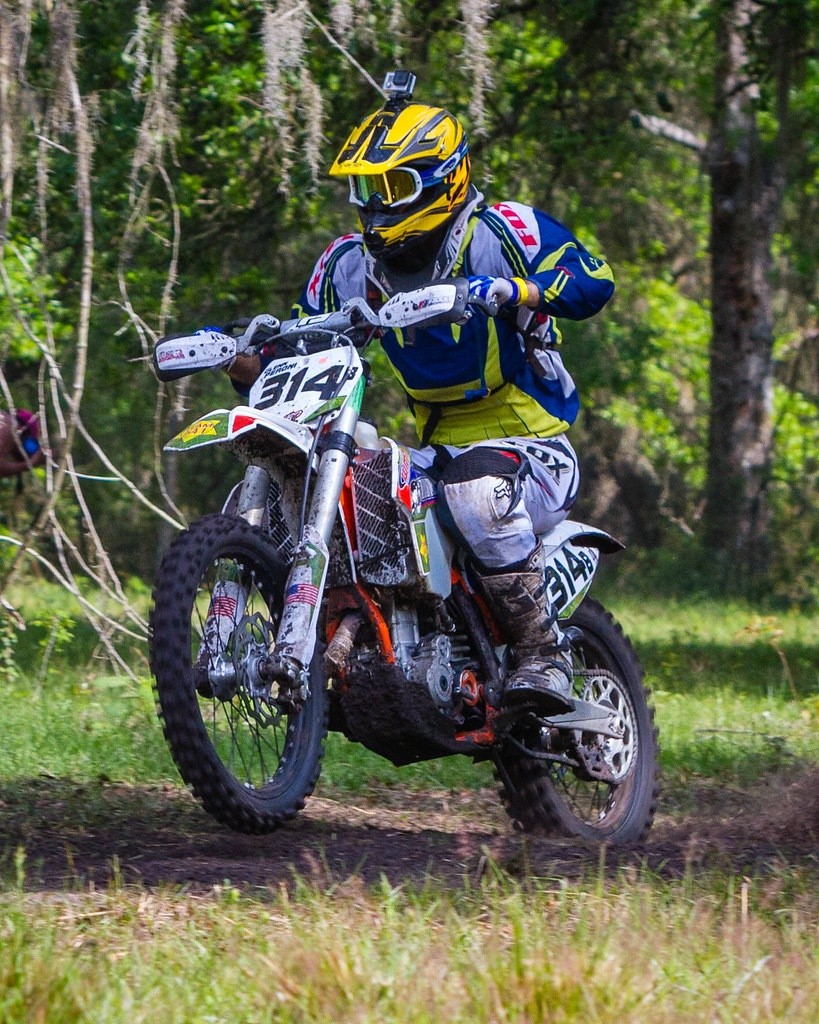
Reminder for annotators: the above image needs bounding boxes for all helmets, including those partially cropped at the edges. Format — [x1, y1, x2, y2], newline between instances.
[328, 103, 471, 275]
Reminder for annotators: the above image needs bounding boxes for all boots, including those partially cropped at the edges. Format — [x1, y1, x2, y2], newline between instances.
[470, 537, 576, 718]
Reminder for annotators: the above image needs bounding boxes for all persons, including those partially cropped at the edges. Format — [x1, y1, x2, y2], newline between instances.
[193, 69, 615, 715]
[0, 408, 46, 478]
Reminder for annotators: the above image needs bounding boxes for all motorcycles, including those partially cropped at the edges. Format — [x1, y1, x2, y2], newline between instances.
[147, 275, 662, 858]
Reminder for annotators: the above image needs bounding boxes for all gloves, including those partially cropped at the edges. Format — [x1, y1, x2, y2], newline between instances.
[194, 327, 232, 373]
[467, 275, 518, 317]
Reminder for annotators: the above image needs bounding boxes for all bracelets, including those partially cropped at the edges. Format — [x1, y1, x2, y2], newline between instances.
[509, 277, 528, 306]
[501, 278, 518, 306]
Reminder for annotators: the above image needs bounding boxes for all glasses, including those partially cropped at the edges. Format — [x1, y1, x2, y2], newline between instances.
[349, 165, 423, 208]
